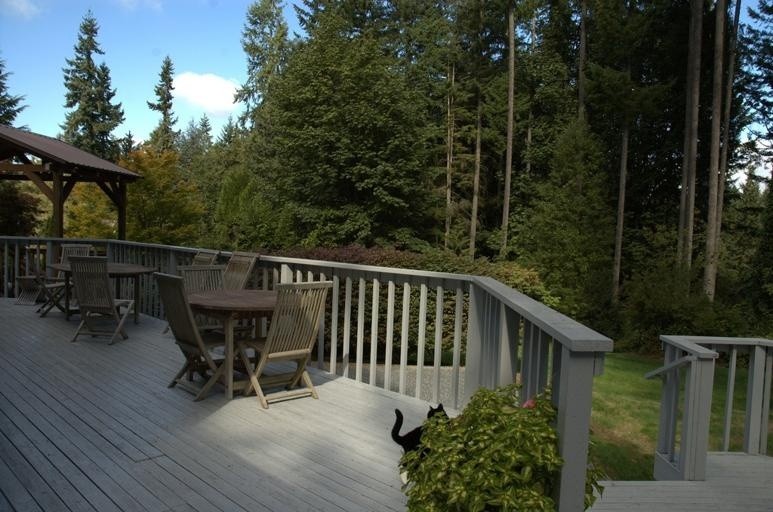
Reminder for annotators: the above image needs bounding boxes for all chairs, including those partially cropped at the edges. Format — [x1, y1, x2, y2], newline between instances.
[66, 256, 135, 345]
[162, 247, 221, 334]
[17, 265, 65, 317]
[153, 271, 250, 402]
[206, 251, 261, 326]
[53, 244, 92, 304]
[236, 281, 334, 408]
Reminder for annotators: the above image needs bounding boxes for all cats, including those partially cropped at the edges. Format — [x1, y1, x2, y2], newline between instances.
[390, 403, 449, 486]
